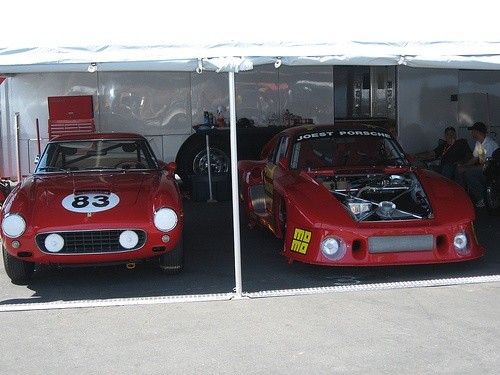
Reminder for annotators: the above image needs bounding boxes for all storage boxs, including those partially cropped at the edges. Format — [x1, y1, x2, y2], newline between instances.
[191, 173, 228, 201]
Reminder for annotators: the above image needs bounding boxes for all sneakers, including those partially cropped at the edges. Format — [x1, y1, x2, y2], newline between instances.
[476, 199, 485, 207]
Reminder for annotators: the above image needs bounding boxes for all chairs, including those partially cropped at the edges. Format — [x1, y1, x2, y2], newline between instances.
[298, 141, 320, 167]
[421, 137, 500, 209]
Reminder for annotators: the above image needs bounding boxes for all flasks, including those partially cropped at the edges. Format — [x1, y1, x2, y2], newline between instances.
[204, 110, 216, 128]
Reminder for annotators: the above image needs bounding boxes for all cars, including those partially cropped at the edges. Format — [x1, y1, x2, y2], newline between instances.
[0, 133, 182, 286]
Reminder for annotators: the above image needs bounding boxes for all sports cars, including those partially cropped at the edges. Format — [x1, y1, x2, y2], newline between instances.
[239, 124, 485, 267]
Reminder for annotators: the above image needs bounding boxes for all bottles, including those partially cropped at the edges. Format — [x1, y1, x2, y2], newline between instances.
[479, 146, 485, 165]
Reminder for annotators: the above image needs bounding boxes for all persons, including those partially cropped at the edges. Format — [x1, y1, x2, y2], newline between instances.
[420, 126, 472, 179]
[453, 121, 499, 208]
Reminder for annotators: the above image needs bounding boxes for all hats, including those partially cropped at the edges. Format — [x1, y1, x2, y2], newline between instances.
[468, 122, 487, 132]
